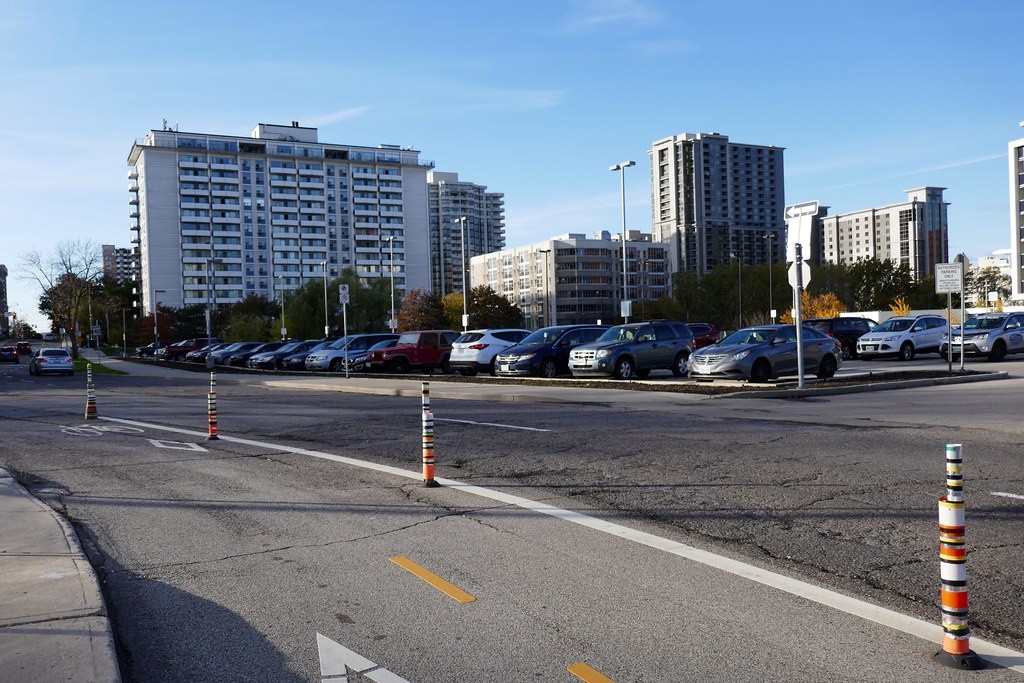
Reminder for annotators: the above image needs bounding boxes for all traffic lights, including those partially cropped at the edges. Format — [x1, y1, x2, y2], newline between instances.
[13, 312, 16, 319]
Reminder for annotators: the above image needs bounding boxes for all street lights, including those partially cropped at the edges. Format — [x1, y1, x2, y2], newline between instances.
[539, 249, 551, 328]
[455, 216, 470, 331]
[385, 236, 397, 333]
[204, 258, 223, 367]
[153, 289, 166, 361]
[610, 160, 637, 324]
[279, 259, 331, 339]
[763, 233, 778, 325]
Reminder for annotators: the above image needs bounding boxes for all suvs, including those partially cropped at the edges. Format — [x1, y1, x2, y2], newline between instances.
[568, 321, 695, 381]
[856, 313, 951, 361]
[367, 330, 462, 373]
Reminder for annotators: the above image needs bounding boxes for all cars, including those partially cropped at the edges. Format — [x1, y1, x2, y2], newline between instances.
[687, 324, 843, 384]
[940, 312, 1024, 363]
[80, 333, 399, 374]
[449, 328, 537, 377]
[802, 310, 876, 360]
[494, 324, 615, 377]
[685, 323, 719, 349]
[0, 333, 75, 377]
